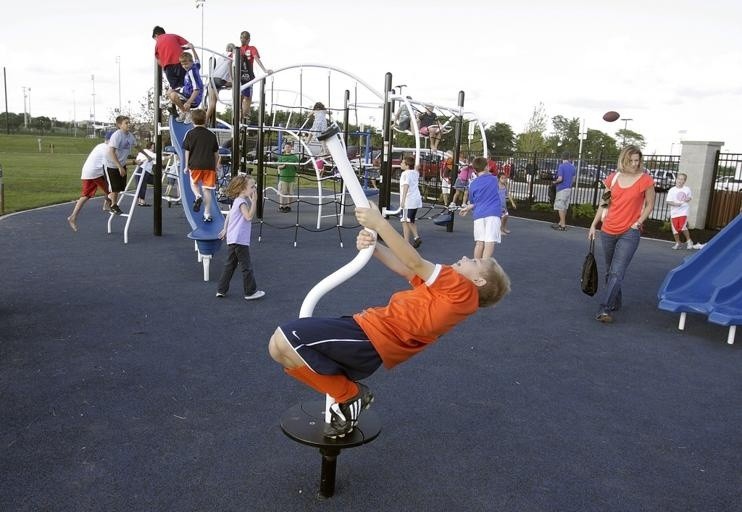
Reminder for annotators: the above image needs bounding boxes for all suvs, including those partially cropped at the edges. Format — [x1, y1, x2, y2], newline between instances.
[714, 174, 742, 194]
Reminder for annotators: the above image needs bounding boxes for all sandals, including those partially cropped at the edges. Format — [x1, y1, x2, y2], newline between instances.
[138, 200, 152, 206]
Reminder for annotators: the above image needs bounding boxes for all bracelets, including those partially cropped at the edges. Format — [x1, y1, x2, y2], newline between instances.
[635, 222, 642, 227]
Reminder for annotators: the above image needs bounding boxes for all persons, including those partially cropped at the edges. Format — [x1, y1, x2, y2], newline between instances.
[666, 172, 693, 250]
[588, 145, 656, 323]
[552, 151, 577, 232]
[269, 201, 512, 440]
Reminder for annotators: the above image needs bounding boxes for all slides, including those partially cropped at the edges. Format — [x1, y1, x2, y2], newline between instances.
[658, 217, 741, 327]
[170, 117, 227, 242]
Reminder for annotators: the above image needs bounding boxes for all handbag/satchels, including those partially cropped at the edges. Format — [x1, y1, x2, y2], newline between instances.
[580, 235, 599, 297]
[601, 190, 613, 226]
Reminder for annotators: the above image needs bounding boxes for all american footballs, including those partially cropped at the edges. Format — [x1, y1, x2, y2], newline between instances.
[603, 111, 620, 122]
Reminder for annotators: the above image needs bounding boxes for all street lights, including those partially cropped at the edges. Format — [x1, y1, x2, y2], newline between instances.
[197, 0, 208, 78]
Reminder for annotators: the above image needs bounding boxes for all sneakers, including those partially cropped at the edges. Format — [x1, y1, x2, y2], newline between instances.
[448, 201, 458, 212]
[551, 222, 569, 231]
[412, 236, 422, 248]
[193, 195, 204, 213]
[202, 215, 213, 223]
[175, 109, 193, 125]
[501, 227, 511, 236]
[245, 289, 267, 300]
[216, 292, 226, 297]
[672, 239, 694, 252]
[103, 199, 124, 215]
[592, 303, 621, 323]
[278, 206, 292, 212]
[323, 380, 374, 440]
[460, 203, 468, 209]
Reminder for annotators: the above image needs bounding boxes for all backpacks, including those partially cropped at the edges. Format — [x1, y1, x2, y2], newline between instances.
[240, 55, 256, 84]
